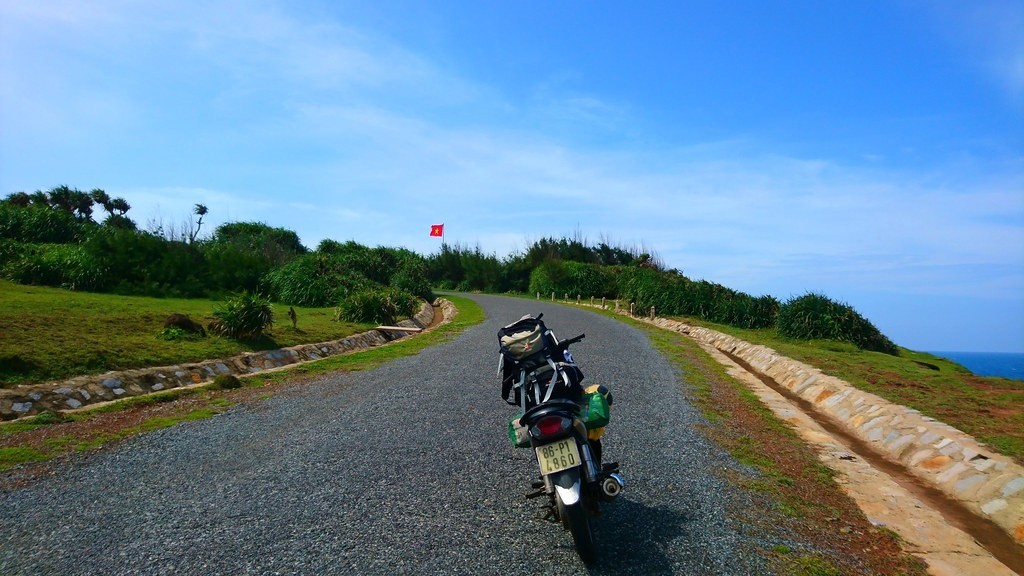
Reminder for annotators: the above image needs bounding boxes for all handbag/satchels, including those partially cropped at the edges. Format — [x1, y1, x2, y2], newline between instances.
[497, 314, 555, 382]
[513, 359, 585, 410]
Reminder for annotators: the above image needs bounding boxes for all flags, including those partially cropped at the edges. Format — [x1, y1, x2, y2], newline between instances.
[429, 223, 443, 237]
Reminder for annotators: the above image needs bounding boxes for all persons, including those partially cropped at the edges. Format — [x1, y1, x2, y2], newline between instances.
[288, 306, 297, 327]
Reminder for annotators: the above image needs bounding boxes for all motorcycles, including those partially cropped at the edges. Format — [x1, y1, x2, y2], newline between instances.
[500, 332, 627, 563]
